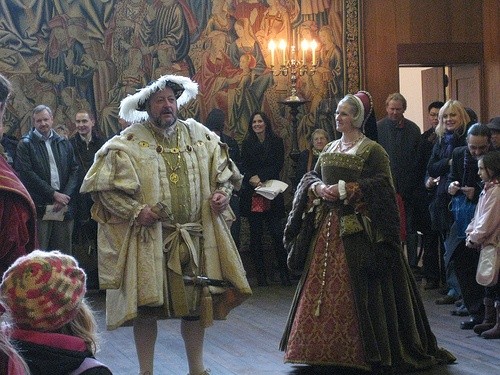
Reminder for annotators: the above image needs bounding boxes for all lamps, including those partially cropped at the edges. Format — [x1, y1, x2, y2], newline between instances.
[268, 37, 318, 196]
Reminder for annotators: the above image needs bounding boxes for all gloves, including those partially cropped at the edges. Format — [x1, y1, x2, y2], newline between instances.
[134, 201, 174, 243]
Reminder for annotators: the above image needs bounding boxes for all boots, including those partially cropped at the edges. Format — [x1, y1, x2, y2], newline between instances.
[482, 301, 500, 339]
[473, 298, 495, 336]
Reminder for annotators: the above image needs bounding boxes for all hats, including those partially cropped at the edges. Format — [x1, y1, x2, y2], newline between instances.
[487, 117, 500, 132]
[117, 74, 199, 123]
[348, 91, 373, 133]
[0, 249, 87, 332]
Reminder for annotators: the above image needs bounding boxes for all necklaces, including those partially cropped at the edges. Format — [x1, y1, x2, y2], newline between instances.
[146, 122, 181, 184]
[337, 133, 364, 153]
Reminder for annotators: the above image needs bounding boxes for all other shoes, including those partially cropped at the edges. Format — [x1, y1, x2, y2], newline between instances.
[256, 270, 270, 286]
[450, 306, 470, 316]
[435, 293, 461, 304]
[280, 269, 292, 286]
[460, 314, 481, 329]
[424, 279, 442, 290]
[456, 300, 463, 307]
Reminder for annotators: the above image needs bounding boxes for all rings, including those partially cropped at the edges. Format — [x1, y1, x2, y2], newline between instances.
[323, 197, 325, 201]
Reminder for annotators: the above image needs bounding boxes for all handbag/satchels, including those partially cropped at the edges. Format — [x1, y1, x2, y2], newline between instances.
[476, 245, 499, 287]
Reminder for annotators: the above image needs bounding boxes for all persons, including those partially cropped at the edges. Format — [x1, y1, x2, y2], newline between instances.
[79, 75, 252, 375]
[240, 111, 291, 287]
[15, 105, 81, 256]
[0, 76, 38, 281]
[278, 92, 457, 375]
[374, 92, 421, 276]
[422, 99, 500, 338]
[0, 249, 112, 375]
[69, 108, 108, 289]
[205, 108, 243, 254]
[294, 128, 331, 187]
